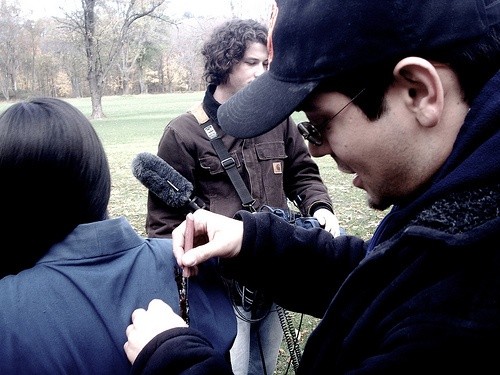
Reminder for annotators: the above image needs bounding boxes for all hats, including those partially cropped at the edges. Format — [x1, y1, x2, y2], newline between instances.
[217, 1, 500, 138]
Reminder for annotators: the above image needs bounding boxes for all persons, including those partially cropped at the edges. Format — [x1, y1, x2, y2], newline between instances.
[146, 17, 340, 375]
[0, 97, 239, 375]
[171, 0, 500, 375]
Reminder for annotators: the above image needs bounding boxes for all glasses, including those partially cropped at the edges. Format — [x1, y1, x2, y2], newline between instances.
[297, 78, 377, 146]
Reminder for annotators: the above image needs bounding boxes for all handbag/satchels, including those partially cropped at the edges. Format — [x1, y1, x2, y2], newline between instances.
[258, 206, 346, 239]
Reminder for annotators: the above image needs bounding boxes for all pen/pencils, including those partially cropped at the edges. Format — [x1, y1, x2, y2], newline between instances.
[175, 213, 196, 329]
